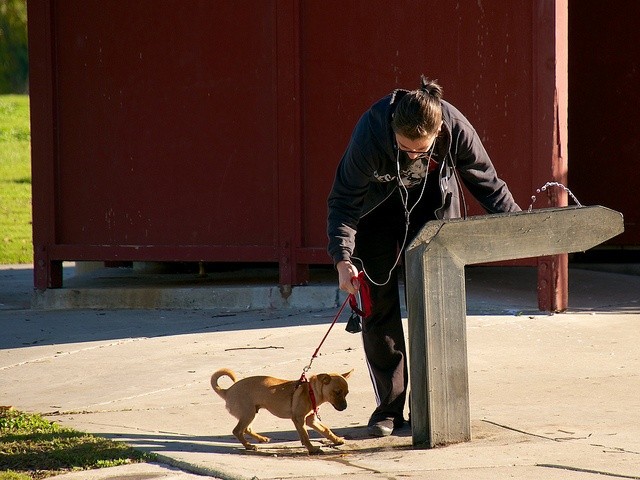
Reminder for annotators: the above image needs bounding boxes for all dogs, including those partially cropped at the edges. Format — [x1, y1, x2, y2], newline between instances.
[208, 367, 354, 454]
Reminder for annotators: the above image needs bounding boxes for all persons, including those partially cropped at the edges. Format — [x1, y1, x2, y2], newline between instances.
[327, 77, 522, 437]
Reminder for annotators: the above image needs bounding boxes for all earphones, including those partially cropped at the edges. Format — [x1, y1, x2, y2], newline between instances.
[351, 127, 441, 286]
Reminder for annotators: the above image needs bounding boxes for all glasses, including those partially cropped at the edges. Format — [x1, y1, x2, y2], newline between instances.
[393, 132, 436, 153]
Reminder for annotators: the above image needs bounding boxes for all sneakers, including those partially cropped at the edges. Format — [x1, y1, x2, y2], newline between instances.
[368, 416, 395, 436]
